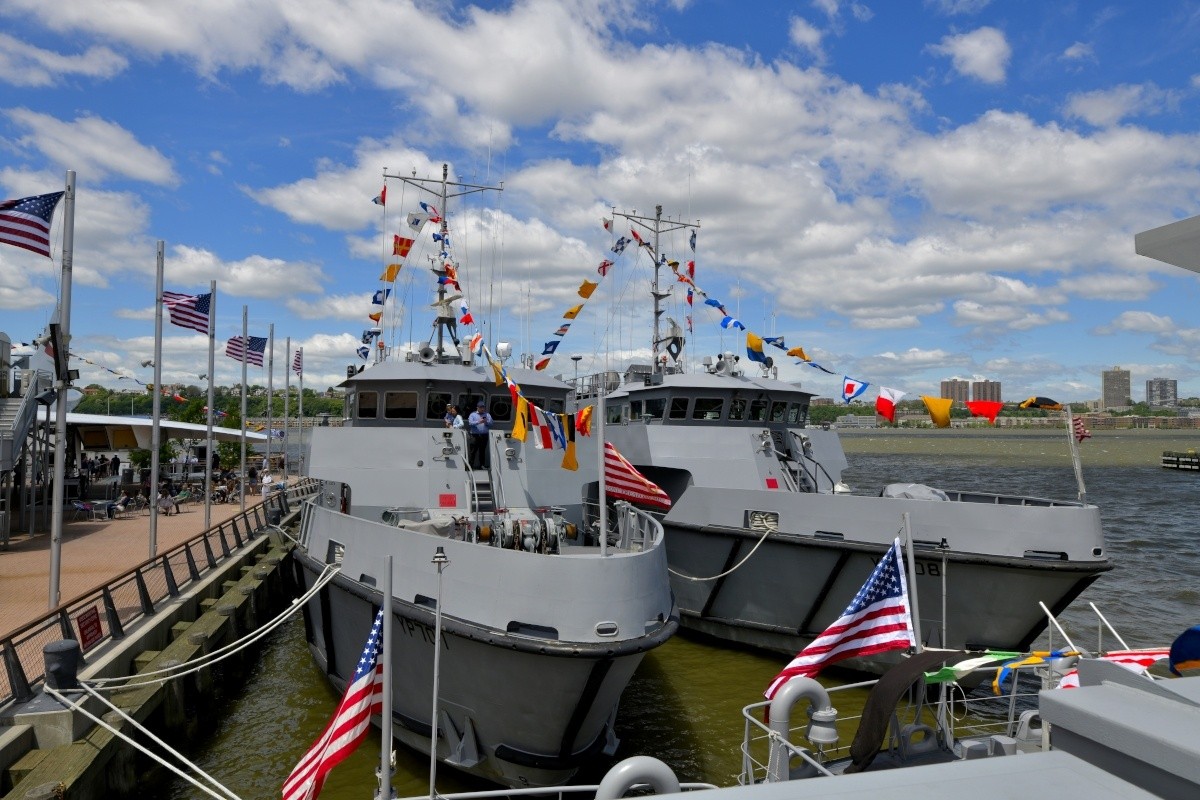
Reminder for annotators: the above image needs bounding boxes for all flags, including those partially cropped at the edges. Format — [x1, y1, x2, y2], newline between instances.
[281, 607, 383, 800]
[841, 376, 869, 405]
[371, 182, 387, 207]
[0, 191, 65, 260]
[162, 291, 212, 335]
[604, 440, 671, 509]
[1017, 396, 1063, 411]
[919, 395, 954, 428]
[875, 385, 906, 423]
[763, 537, 918, 724]
[43, 300, 61, 365]
[923, 646, 1170, 690]
[67, 351, 267, 433]
[225, 335, 268, 367]
[356, 201, 839, 471]
[292, 350, 301, 376]
[1072, 417, 1091, 444]
[966, 399, 1004, 423]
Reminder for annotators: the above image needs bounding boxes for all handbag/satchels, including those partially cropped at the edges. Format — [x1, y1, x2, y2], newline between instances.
[249, 482, 251, 486]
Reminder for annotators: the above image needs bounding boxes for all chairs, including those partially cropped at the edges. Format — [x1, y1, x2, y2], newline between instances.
[115, 497, 149, 521]
[158, 492, 192, 518]
[70, 500, 90, 522]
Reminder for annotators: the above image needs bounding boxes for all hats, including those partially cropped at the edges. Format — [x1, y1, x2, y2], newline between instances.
[264, 469, 268, 472]
[114, 453, 118, 456]
[192, 455, 196, 457]
[101, 453, 105, 456]
[477, 401, 486, 408]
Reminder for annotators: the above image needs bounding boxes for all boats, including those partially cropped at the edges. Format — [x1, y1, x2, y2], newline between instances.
[562, 201, 1118, 708]
[288, 161, 682, 800]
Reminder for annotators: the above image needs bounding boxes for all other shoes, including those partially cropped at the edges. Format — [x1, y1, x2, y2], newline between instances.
[104, 518, 111, 521]
[479, 465, 487, 470]
[103, 516, 108, 518]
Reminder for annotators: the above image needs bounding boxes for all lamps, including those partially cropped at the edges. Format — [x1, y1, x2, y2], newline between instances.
[442, 447, 453, 455]
[802, 441, 812, 449]
[761, 440, 771, 447]
[505, 448, 515, 457]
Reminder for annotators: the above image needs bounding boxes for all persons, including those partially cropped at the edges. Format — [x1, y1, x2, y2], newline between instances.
[12, 450, 285, 521]
[444, 404, 464, 429]
[466, 401, 493, 471]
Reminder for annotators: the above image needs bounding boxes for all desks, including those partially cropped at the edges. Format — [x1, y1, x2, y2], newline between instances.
[90, 500, 114, 521]
